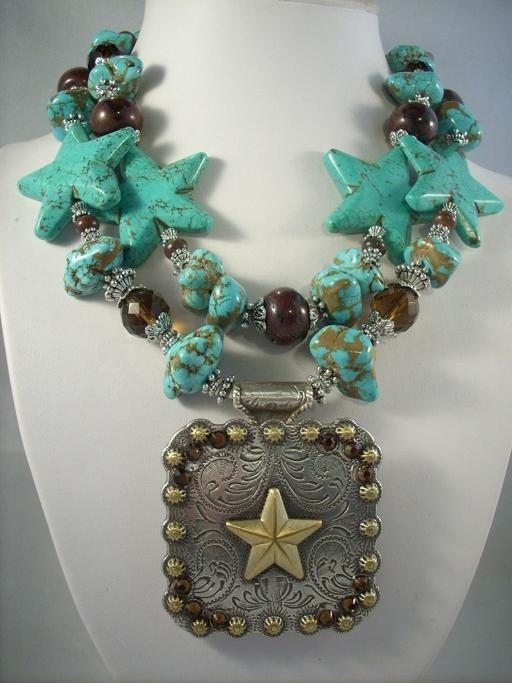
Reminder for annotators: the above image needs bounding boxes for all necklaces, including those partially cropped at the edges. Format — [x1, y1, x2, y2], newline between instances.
[19, 30, 503, 638]
